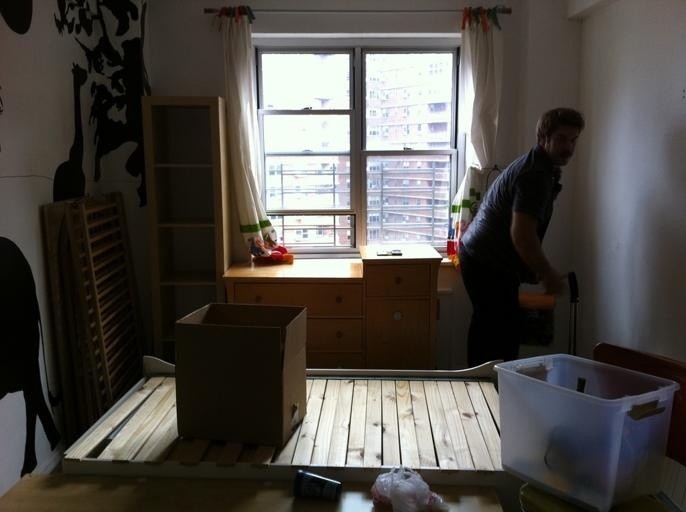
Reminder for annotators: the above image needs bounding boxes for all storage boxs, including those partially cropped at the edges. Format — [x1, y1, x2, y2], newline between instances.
[492, 352, 682, 511]
[172, 301, 308, 449]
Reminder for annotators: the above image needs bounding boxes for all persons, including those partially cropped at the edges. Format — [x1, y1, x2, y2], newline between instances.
[456, 108, 588, 367]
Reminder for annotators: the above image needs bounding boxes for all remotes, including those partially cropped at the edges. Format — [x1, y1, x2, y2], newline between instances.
[378, 248, 402, 256]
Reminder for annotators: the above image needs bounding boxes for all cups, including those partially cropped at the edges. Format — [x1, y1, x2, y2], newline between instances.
[292, 470, 342, 503]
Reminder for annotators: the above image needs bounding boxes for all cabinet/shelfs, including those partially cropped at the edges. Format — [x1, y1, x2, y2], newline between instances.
[359, 242, 444, 370]
[140, 94, 233, 362]
[222, 258, 363, 368]
